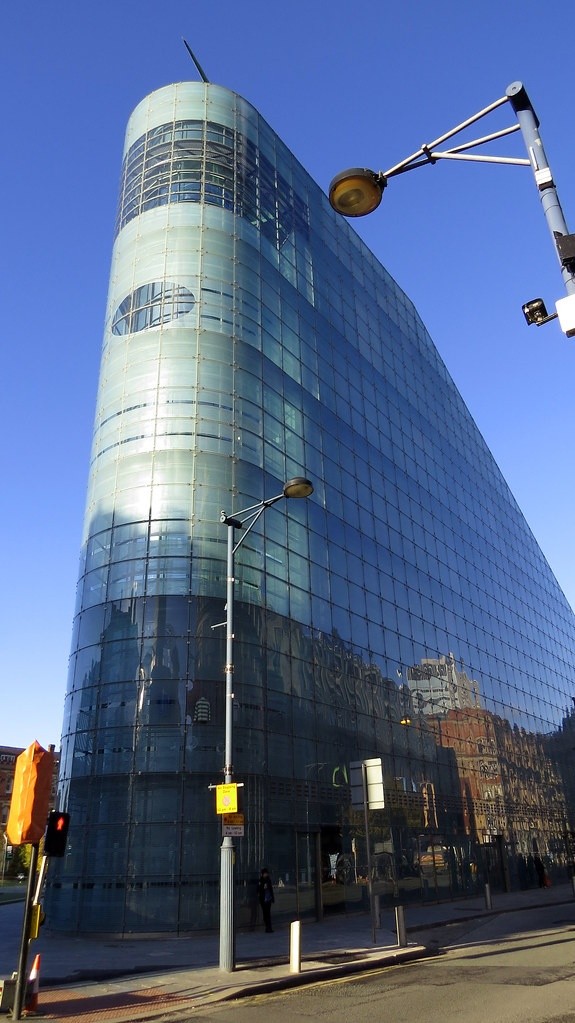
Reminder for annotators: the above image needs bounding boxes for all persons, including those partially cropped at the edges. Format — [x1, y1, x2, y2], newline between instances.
[557, 855, 562, 867]
[256, 868, 275, 934]
[246, 873, 259, 933]
[542, 852, 553, 869]
[517, 852, 534, 891]
[533, 854, 546, 889]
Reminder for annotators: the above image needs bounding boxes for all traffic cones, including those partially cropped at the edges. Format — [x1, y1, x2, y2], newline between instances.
[23, 952, 43, 1013]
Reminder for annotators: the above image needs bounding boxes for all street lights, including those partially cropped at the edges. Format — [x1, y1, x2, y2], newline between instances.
[326, 81, 575, 336]
[213, 473, 319, 972]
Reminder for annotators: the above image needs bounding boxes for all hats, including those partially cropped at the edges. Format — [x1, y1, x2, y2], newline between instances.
[261, 868, 269, 876]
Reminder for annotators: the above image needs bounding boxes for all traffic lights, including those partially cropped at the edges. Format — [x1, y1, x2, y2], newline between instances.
[41, 810, 70, 858]
